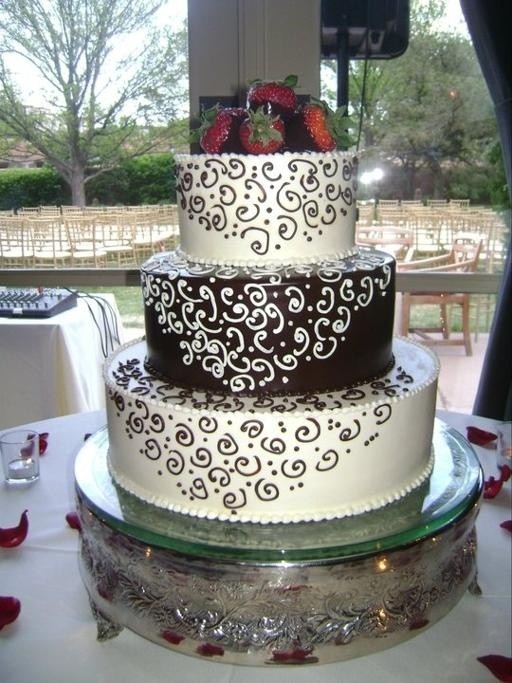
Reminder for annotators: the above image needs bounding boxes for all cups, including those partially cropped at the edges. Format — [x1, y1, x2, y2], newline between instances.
[496, 421, 512, 473]
[0, 428, 41, 488]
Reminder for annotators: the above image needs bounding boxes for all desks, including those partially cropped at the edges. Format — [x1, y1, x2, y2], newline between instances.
[0, 407, 512, 683]
[0, 293, 127, 429]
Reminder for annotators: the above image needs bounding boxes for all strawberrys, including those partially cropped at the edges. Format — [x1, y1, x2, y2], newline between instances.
[198, 80, 337, 154]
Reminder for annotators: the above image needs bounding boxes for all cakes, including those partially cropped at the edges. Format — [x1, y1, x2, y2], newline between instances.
[101, 152, 441, 526]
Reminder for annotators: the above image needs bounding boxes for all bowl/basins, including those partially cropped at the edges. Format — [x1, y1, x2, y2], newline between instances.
[71, 474, 484, 667]
